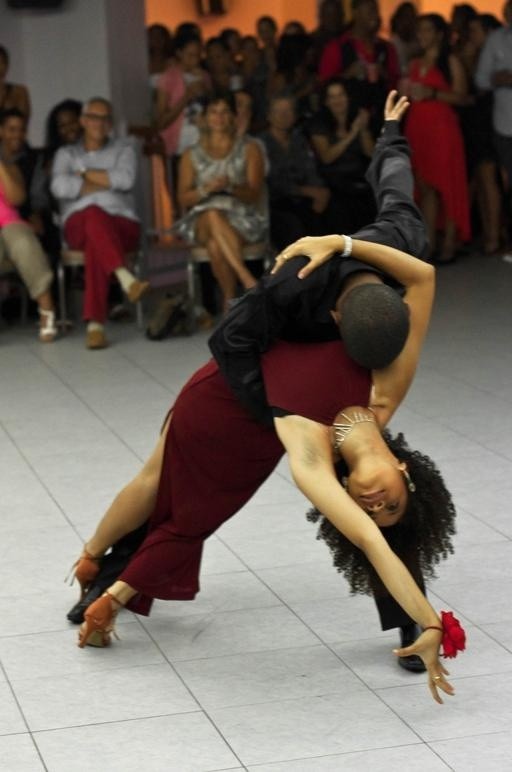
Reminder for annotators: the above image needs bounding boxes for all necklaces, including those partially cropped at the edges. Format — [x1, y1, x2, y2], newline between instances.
[333, 409, 376, 450]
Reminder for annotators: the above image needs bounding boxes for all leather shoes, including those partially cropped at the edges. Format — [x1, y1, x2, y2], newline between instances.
[107, 305, 131, 321]
[127, 280, 151, 303]
[86, 329, 106, 349]
[398, 622, 427, 672]
[67, 582, 104, 625]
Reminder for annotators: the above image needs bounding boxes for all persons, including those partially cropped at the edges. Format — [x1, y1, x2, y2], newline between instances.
[63, 233, 458, 707]
[1, 1, 511, 348]
[65, 89, 426, 674]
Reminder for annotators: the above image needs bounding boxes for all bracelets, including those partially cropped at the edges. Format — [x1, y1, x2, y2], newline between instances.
[423, 611, 466, 660]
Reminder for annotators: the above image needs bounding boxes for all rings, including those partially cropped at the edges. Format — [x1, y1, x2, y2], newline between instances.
[433, 676, 441, 680]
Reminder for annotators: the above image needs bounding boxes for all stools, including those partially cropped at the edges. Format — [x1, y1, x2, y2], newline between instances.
[57, 228, 145, 334]
[187, 220, 274, 325]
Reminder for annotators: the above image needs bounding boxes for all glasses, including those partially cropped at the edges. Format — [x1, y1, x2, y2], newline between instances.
[83, 113, 112, 122]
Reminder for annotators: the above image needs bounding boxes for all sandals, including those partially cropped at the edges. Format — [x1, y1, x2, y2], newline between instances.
[36, 302, 59, 342]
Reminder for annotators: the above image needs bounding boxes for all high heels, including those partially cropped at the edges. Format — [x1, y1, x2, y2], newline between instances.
[75, 549, 102, 605]
[79, 589, 125, 649]
